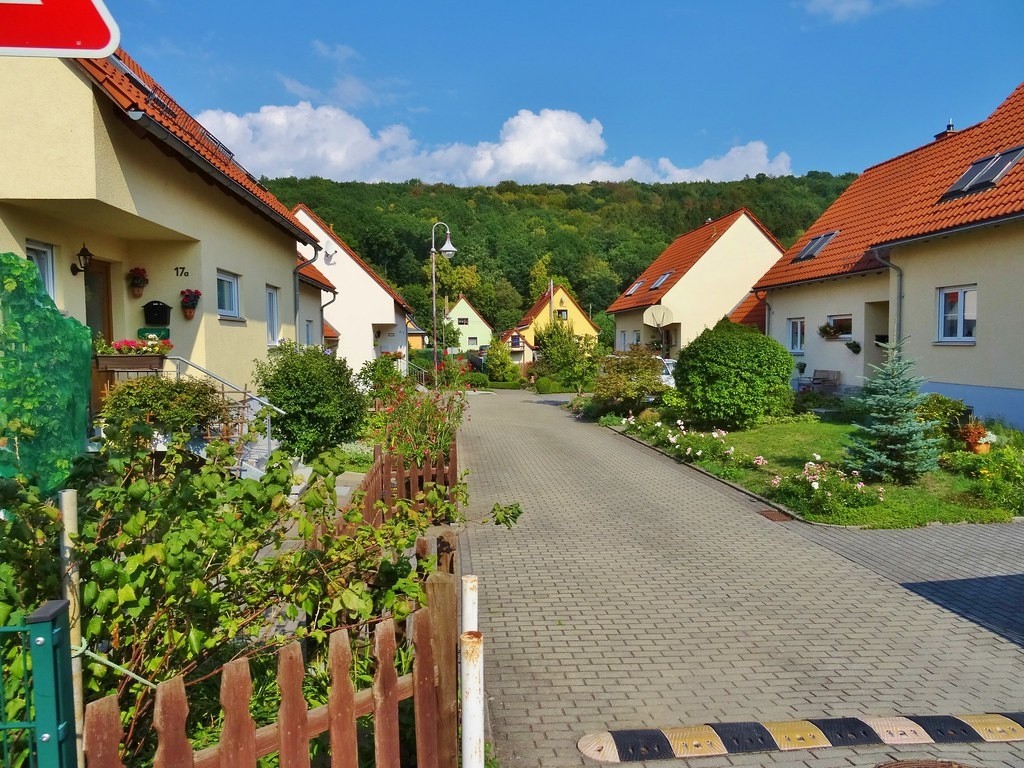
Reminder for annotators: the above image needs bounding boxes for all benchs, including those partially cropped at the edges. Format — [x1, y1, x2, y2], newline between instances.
[796, 369, 840, 393]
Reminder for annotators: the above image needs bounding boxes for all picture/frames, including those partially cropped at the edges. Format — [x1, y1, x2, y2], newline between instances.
[184, 308, 195, 319]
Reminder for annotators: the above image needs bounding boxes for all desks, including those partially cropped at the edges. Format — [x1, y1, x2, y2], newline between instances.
[803, 377, 828, 391]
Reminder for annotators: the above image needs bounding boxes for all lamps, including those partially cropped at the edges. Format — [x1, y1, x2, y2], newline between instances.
[375, 330, 381, 338]
[71, 243, 94, 276]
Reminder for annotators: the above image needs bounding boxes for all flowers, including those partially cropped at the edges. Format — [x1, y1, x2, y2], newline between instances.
[816, 322, 842, 338]
[127, 267, 148, 287]
[180, 288, 202, 309]
[954, 416, 997, 443]
[94, 331, 175, 354]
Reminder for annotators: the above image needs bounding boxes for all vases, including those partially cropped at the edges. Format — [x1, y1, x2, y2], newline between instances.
[97, 354, 165, 368]
[827, 335, 839, 339]
[966, 442, 991, 454]
[130, 287, 143, 297]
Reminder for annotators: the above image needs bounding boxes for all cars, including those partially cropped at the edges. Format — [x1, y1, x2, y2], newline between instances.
[630, 356, 675, 404]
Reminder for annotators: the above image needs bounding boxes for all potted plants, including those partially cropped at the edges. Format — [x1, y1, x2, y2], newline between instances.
[793, 362, 806, 373]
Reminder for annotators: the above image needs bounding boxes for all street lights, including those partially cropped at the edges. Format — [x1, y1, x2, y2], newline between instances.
[430, 222, 457, 394]
